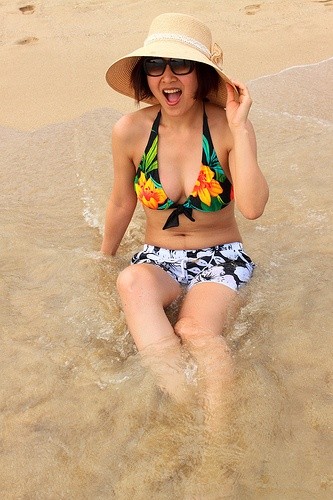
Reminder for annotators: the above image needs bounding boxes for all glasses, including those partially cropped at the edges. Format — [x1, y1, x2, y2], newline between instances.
[143, 57, 196, 77]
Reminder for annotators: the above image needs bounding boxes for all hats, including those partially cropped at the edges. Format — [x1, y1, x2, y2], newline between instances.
[105, 13, 240, 108]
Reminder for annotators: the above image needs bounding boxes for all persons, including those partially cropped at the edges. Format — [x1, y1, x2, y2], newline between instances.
[100, 11, 270, 428]
